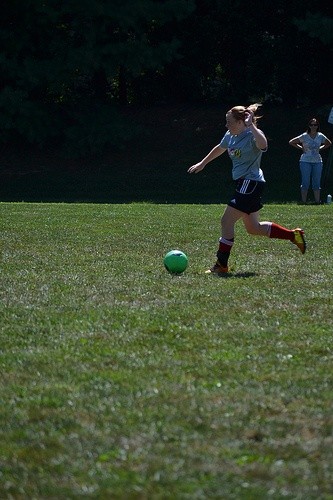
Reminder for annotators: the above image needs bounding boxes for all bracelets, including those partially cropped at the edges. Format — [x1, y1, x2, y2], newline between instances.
[247, 122, 253, 128]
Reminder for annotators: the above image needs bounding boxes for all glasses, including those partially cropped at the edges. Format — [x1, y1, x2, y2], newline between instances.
[310, 124, 317, 127]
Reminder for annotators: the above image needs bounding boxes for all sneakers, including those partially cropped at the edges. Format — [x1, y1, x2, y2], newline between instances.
[292, 228, 307, 254]
[205, 262, 228, 274]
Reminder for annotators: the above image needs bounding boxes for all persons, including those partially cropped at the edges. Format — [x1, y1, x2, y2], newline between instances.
[289, 119, 332, 205]
[187, 103, 306, 274]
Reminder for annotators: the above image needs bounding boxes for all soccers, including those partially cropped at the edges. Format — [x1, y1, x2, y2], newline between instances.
[164, 250, 188, 275]
